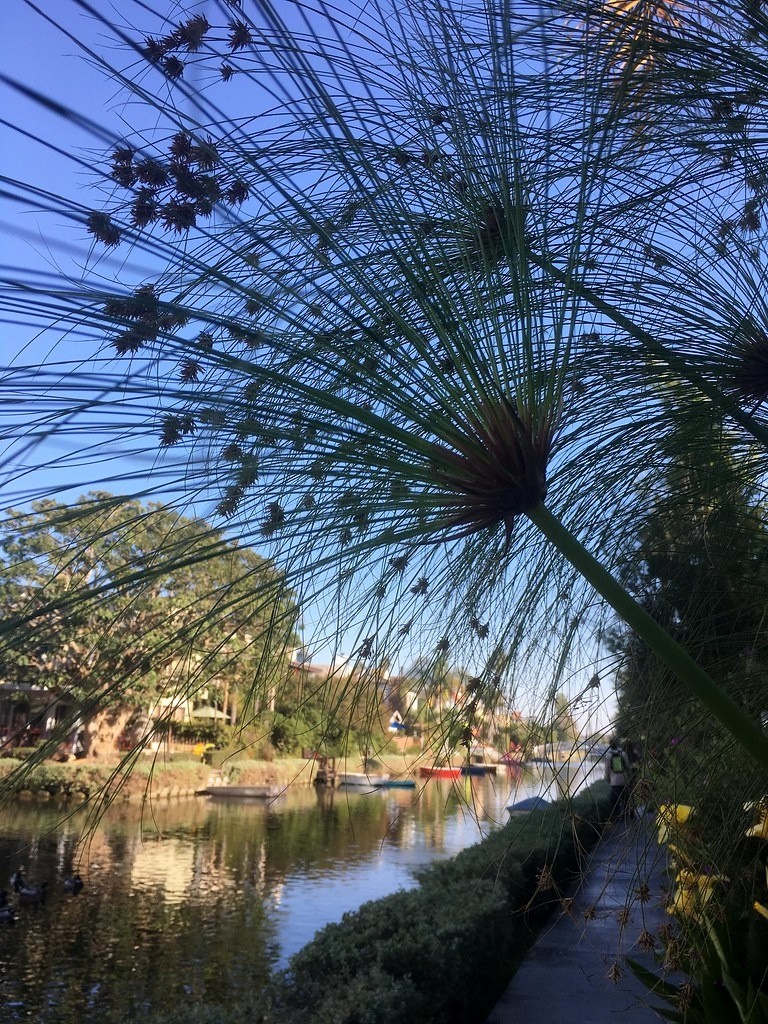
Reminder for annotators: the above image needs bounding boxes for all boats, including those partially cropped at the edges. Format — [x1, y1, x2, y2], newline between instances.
[386, 779, 415, 788]
[207, 784, 287, 798]
[337, 771, 390, 786]
[420, 762, 497, 777]
